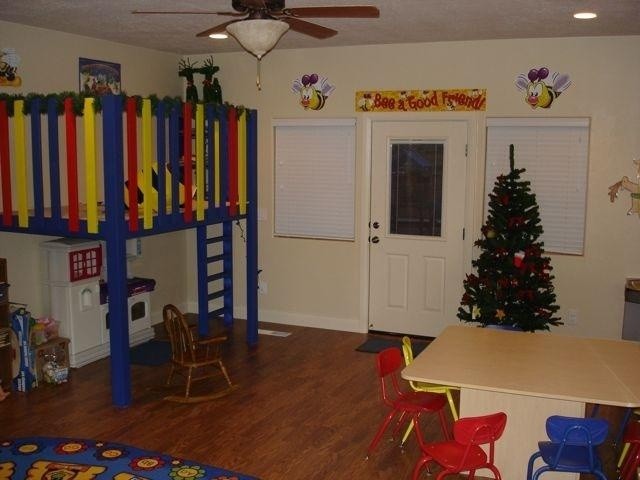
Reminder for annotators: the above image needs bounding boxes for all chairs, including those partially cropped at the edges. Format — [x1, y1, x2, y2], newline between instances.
[367, 349, 454, 460]
[159, 302, 234, 403]
[524, 413, 612, 480]
[409, 411, 507, 480]
[392, 336, 462, 449]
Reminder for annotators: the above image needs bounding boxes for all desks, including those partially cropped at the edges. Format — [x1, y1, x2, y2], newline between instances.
[402, 326, 640, 480]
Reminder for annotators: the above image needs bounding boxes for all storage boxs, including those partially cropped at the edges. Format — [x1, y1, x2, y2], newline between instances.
[25, 317, 60, 343]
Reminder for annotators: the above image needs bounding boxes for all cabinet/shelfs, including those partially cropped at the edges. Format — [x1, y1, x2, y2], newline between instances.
[100, 292, 150, 340]
[48, 246, 103, 354]
[0, 259, 14, 400]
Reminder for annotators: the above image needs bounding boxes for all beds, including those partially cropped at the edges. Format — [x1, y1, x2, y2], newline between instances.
[1, 95, 261, 408]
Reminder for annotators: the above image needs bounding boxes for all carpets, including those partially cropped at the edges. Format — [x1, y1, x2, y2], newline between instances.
[0, 437, 259, 479]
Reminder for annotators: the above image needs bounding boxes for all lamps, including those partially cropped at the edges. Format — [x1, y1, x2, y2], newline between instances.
[221, 17, 292, 92]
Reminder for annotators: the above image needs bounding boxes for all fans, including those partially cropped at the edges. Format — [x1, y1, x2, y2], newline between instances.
[125, 1, 380, 40]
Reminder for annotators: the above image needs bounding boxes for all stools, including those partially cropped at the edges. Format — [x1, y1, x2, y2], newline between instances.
[31, 338, 72, 383]
